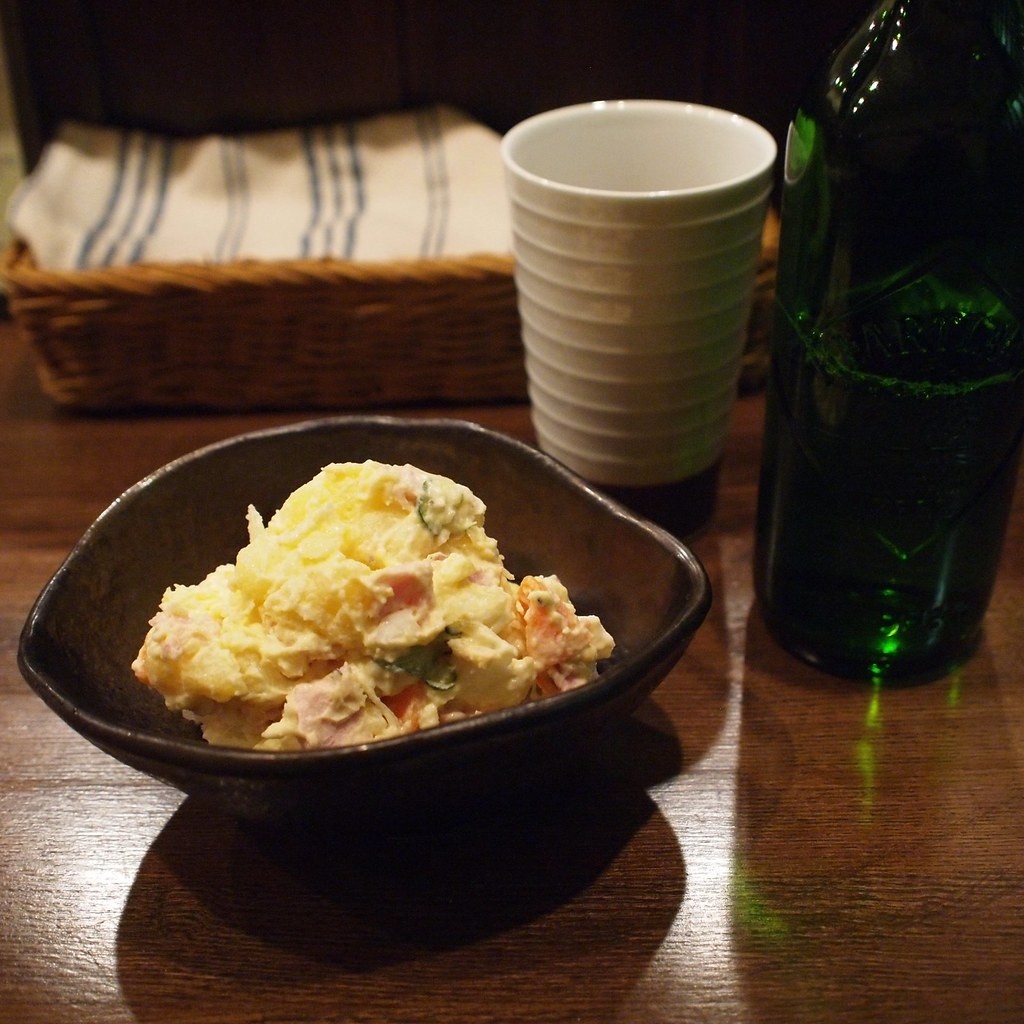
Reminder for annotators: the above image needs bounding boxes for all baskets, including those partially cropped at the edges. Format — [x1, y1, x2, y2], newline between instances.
[0, 125, 787, 411]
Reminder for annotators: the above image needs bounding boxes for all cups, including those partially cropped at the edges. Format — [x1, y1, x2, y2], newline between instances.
[499, 98, 778, 489]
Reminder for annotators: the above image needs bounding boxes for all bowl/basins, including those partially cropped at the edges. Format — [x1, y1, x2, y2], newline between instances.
[16, 415, 714, 825]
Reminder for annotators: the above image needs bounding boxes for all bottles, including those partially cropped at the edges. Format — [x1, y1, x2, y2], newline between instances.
[749, 0, 1024, 691]
